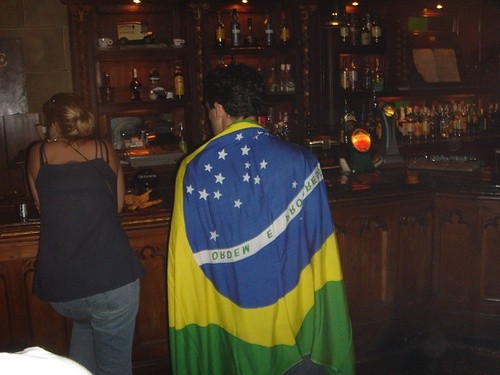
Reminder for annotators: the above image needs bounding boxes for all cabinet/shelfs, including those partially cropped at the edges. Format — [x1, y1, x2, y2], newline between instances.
[67, 0, 500, 188]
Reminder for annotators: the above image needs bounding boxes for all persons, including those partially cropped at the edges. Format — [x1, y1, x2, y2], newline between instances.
[164, 65, 356, 375]
[27, 93, 141, 375]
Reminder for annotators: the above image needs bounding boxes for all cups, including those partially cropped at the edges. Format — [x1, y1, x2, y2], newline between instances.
[98, 38, 113, 47]
[173, 38, 185, 45]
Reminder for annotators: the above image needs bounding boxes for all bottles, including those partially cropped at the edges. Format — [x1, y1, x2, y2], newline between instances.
[99, 60, 189, 156]
[390, 96, 500, 142]
[337, 11, 386, 147]
[214, 7, 300, 143]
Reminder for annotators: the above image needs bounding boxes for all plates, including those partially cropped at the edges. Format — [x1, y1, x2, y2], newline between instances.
[97, 46, 112, 50]
[169, 44, 186, 48]
